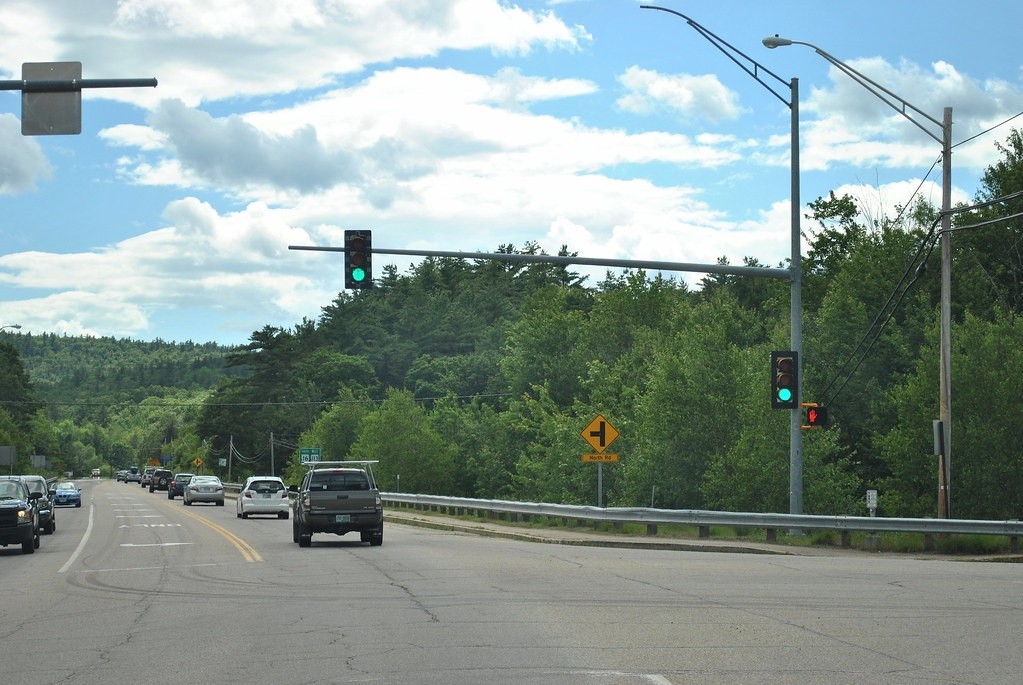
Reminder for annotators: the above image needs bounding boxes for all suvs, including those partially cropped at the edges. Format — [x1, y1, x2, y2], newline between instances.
[148, 468, 174, 493]
[124, 467, 141, 484]
[0, 479, 43, 553]
[289, 461, 385, 548]
[140, 468, 157, 488]
[91, 469, 100, 479]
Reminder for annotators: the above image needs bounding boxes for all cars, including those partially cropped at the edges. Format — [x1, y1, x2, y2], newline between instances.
[116, 470, 128, 482]
[0, 475, 57, 534]
[183, 475, 225, 506]
[168, 474, 195, 500]
[48, 482, 82, 507]
[234, 475, 290, 520]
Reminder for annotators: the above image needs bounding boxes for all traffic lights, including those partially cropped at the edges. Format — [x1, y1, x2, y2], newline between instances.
[770, 351, 799, 410]
[344, 230, 372, 289]
[807, 405, 828, 425]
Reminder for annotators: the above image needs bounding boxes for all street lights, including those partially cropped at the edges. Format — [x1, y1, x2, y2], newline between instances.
[762, 33, 953, 519]
